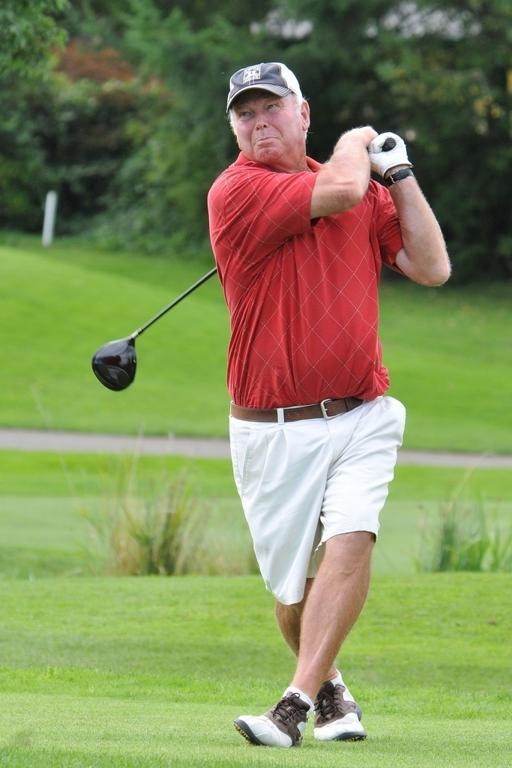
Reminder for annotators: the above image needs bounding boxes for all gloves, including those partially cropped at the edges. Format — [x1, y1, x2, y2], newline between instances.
[369, 132, 412, 179]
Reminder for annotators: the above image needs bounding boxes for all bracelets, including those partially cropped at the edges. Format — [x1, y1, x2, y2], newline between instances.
[385, 168, 415, 188]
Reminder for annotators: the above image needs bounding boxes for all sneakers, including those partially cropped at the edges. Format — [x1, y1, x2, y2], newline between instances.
[234, 671, 366, 748]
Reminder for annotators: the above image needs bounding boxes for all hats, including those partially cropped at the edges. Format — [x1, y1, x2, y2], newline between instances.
[226, 61, 302, 113]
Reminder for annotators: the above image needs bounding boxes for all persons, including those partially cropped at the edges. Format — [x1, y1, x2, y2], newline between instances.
[206, 60, 455, 751]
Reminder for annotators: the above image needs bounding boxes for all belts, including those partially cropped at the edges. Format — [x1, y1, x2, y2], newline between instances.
[230, 397, 362, 423]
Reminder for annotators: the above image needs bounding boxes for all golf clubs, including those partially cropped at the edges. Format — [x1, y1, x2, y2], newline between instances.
[91, 136, 397, 392]
